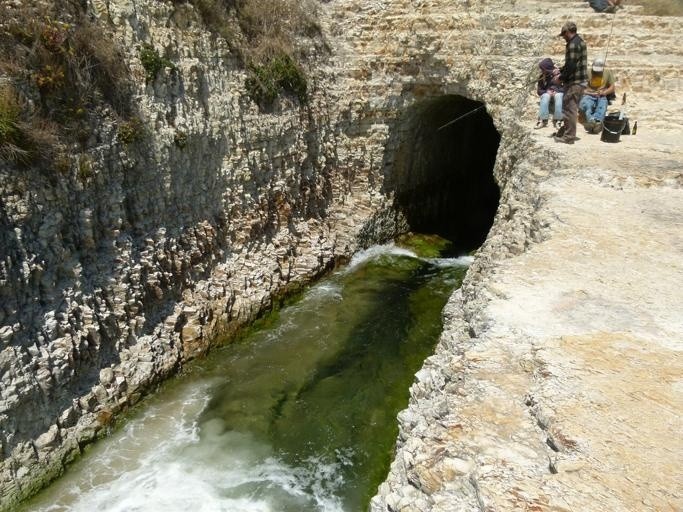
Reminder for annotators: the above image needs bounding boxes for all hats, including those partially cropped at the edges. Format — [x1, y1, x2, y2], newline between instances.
[592, 57, 606, 72]
[559, 21, 575, 36]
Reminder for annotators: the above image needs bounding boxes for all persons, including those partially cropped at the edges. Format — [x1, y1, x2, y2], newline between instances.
[548, 21, 588, 144]
[534, 58, 565, 129]
[578, 53, 615, 134]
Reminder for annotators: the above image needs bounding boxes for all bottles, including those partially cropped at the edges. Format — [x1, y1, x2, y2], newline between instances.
[632, 121, 638, 135]
[621, 93, 627, 105]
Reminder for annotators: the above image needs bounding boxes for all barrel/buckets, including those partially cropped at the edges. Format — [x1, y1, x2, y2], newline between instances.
[600, 114, 628, 142]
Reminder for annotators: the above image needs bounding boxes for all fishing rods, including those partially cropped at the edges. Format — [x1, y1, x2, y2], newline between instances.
[437, 77, 545, 131]
[591, 13, 616, 114]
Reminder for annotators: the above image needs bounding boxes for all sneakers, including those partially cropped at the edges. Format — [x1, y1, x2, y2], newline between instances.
[553, 135, 574, 144]
[555, 121, 562, 130]
[534, 120, 547, 128]
[593, 122, 602, 134]
[582, 121, 594, 134]
